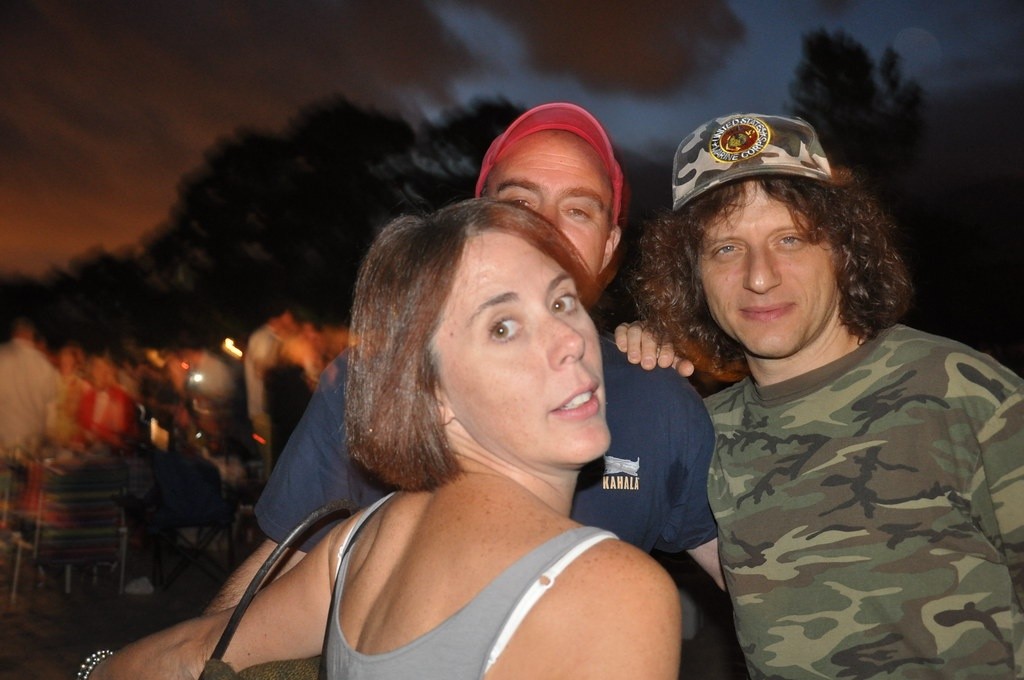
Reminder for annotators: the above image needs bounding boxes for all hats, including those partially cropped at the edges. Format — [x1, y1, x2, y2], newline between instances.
[671, 113, 833, 212]
[475, 101, 624, 224]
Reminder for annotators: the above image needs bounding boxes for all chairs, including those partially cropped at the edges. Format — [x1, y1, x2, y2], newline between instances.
[8, 458, 268, 604]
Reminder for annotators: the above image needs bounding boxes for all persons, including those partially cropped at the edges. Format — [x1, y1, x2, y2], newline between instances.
[201, 104, 728, 617]
[1, 310, 353, 529]
[74, 198, 682, 680]
[614, 113, 1023, 680]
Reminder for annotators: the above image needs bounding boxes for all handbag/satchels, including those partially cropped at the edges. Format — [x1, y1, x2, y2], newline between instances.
[197, 496, 380, 680]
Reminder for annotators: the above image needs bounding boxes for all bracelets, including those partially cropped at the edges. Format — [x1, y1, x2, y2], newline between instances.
[77, 649, 113, 680]
